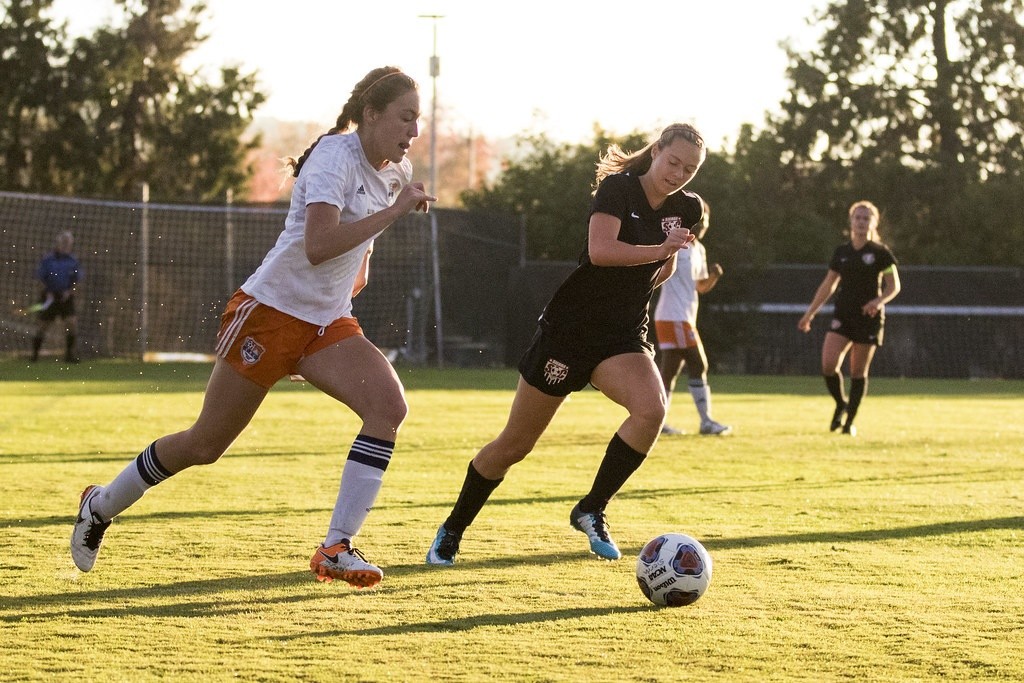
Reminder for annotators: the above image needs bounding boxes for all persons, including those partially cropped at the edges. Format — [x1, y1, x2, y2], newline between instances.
[426, 124, 708, 563]
[71, 65, 435, 588]
[798, 204, 901, 432]
[655, 206, 732, 434]
[32, 232, 81, 363]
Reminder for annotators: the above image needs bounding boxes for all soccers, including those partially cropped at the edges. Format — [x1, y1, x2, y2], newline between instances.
[635, 533, 713, 608]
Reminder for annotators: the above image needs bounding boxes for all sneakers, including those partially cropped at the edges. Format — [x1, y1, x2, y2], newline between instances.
[699, 417, 728, 434]
[309, 538, 383, 590]
[829, 401, 852, 434]
[426, 525, 460, 565]
[569, 499, 621, 559]
[70, 484, 114, 572]
[660, 423, 686, 436]
